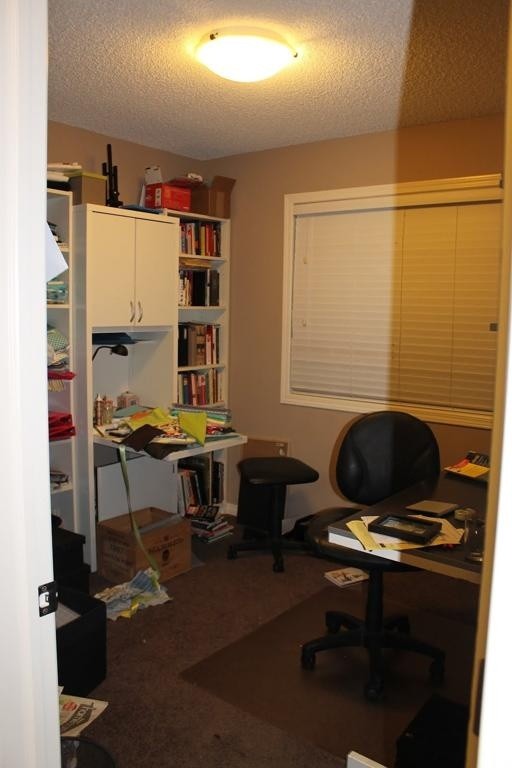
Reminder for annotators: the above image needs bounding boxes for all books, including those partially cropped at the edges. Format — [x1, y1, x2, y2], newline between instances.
[175, 456, 235, 546]
[165, 221, 233, 429]
[323, 567, 369, 588]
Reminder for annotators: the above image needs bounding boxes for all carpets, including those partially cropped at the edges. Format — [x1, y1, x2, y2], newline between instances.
[175, 570, 479, 768]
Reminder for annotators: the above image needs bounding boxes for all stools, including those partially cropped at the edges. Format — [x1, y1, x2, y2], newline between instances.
[226, 454, 314, 573]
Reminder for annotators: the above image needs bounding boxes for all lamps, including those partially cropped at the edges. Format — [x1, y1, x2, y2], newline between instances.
[195, 35, 299, 84]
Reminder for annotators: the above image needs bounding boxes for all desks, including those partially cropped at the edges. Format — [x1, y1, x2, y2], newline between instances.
[326, 468, 489, 585]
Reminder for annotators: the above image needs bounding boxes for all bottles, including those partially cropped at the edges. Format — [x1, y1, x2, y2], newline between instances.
[95, 392, 113, 426]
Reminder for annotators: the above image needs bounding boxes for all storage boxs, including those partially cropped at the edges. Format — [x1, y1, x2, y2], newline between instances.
[54, 527, 109, 700]
[144, 173, 237, 218]
[94, 504, 192, 585]
[68, 175, 106, 204]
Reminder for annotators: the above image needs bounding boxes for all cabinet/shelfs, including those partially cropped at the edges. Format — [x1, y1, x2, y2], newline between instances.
[47, 185, 246, 573]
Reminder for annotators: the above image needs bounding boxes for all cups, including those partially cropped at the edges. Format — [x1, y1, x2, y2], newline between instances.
[464, 518, 485, 562]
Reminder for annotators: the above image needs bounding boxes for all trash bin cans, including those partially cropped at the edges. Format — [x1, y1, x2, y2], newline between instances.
[60, 736, 116, 768]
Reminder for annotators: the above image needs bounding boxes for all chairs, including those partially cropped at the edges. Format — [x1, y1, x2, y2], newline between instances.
[300, 410, 445, 701]
